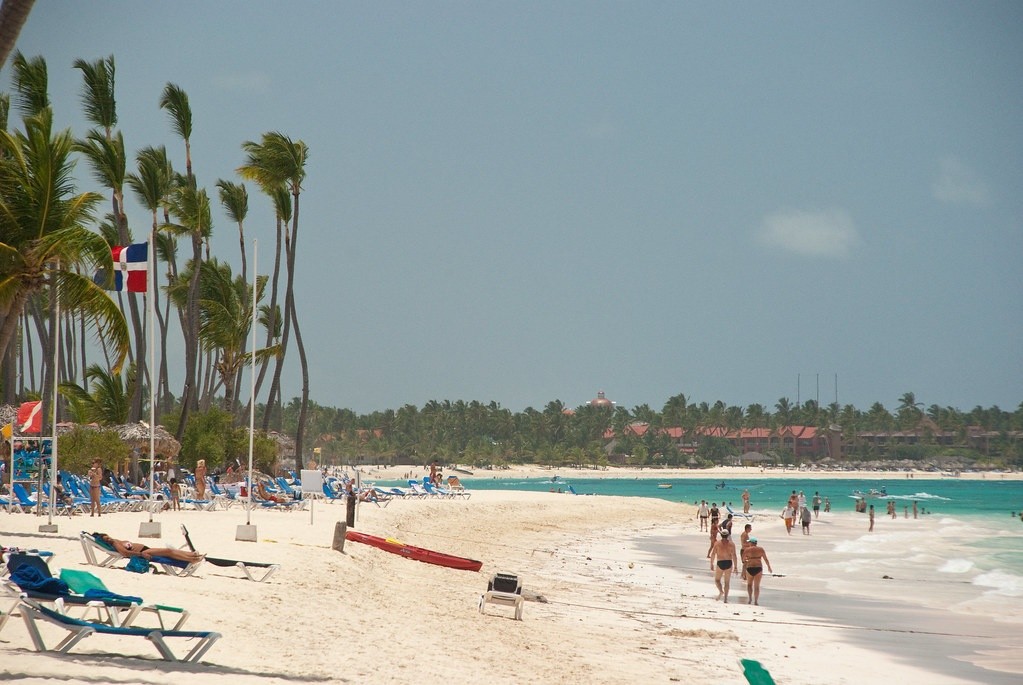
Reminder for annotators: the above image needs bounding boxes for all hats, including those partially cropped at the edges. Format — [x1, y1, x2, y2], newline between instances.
[721, 529, 728, 535]
[747, 538, 758, 543]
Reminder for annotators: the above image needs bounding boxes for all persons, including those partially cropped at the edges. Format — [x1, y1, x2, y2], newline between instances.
[710, 529, 738, 603]
[718, 514, 733, 542]
[707, 517, 718, 558]
[58, 459, 444, 516]
[740, 523, 752, 580]
[742, 538, 773, 606]
[696, 480, 1023, 536]
[102, 533, 200, 562]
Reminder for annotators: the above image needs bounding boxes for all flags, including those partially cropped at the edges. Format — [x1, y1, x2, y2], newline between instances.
[93, 242, 148, 292]
[16, 400, 42, 434]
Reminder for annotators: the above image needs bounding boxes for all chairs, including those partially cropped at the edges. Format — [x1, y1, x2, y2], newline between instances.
[725, 505, 757, 523]
[0, 469, 472, 519]
[0, 523, 280, 664]
[736, 658, 776, 685]
[567, 484, 597, 496]
[477, 572, 524, 621]
